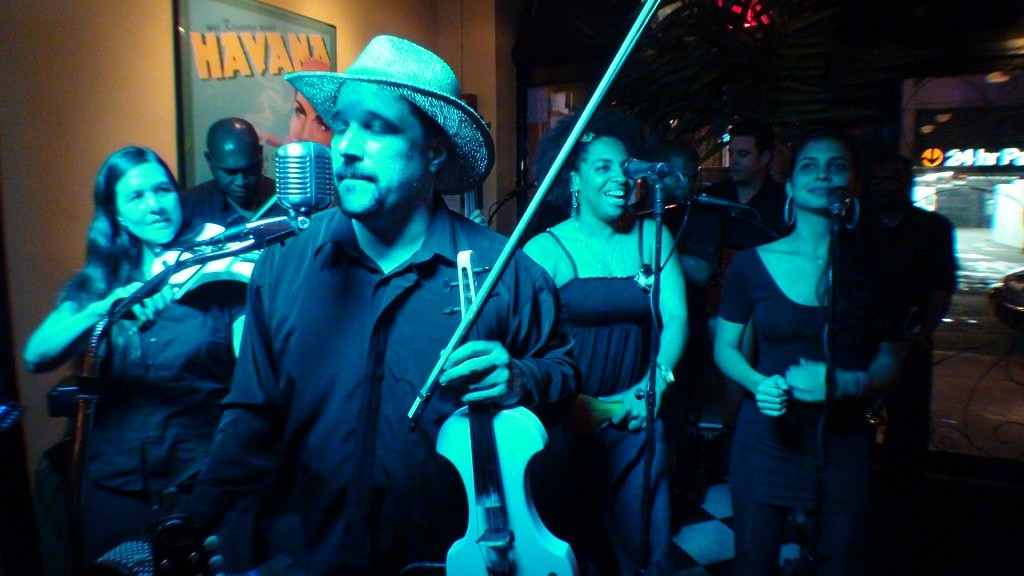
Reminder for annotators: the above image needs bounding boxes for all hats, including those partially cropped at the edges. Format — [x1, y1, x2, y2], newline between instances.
[284, 35, 496, 198]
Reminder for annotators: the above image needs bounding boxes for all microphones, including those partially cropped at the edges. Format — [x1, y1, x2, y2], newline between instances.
[827, 186, 852, 219]
[691, 195, 751, 209]
[621, 158, 672, 181]
[204, 143, 334, 245]
[508, 180, 539, 197]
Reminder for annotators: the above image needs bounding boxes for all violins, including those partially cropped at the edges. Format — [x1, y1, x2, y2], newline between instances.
[433, 248, 577, 576]
[149, 221, 263, 306]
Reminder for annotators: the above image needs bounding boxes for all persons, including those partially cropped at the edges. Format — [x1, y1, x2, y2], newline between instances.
[21, 144, 250, 576]
[181, 115, 289, 234]
[641, 144, 723, 466]
[674, 114, 795, 475]
[177, 35, 585, 576]
[854, 152, 956, 528]
[523, 108, 687, 576]
[711, 127, 908, 576]
[290, 88, 333, 149]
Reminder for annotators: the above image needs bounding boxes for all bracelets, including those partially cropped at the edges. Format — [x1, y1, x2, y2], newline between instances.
[654, 361, 676, 385]
